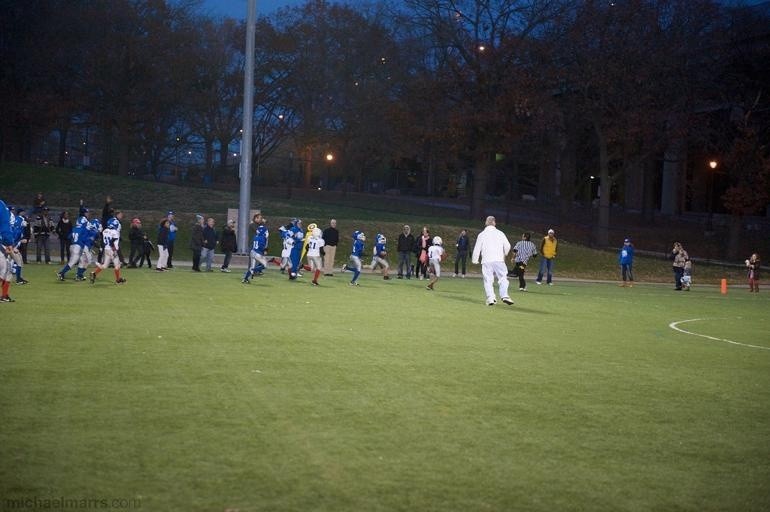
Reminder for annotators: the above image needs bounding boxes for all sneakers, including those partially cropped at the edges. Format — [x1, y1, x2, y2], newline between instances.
[15, 279, 29, 285]
[486, 296, 496, 306]
[241, 257, 436, 290]
[155, 268, 170, 272]
[0, 295, 14, 302]
[503, 298, 513, 306]
[192, 267, 232, 273]
[56, 273, 125, 284]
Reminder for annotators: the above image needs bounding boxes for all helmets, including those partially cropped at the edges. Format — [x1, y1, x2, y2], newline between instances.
[80, 207, 122, 232]
[432, 235, 442, 246]
[256, 218, 323, 239]
[352, 230, 388, 245]
[9, 206, 49, 212]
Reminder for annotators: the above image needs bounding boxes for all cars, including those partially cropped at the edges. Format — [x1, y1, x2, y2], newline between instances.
[330, 182, 387, 193]
[520, 190, 657, 214]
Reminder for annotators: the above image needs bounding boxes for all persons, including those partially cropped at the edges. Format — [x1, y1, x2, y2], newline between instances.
[472, 215, 515, 306]
[536, 227, 558, 286]
[242, 213, 340, 286]
[672, 244, 693, 291]
[342, 231, 366, 286]
[506, 232, 538, 293]
[745, 254, 760, 292]
[452, 229, 469, 279]
[619, 239, 634, 286]
[189, 215, 238, 273]
[362, 233, 391, 280]
[0, 191, 179, 303]
[395, 223, 445, 289]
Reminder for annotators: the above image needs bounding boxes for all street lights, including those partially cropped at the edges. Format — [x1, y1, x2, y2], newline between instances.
[325, 154, 335, 190]
[175, 136, 179, 184]
[706, 156, 721, 231]
